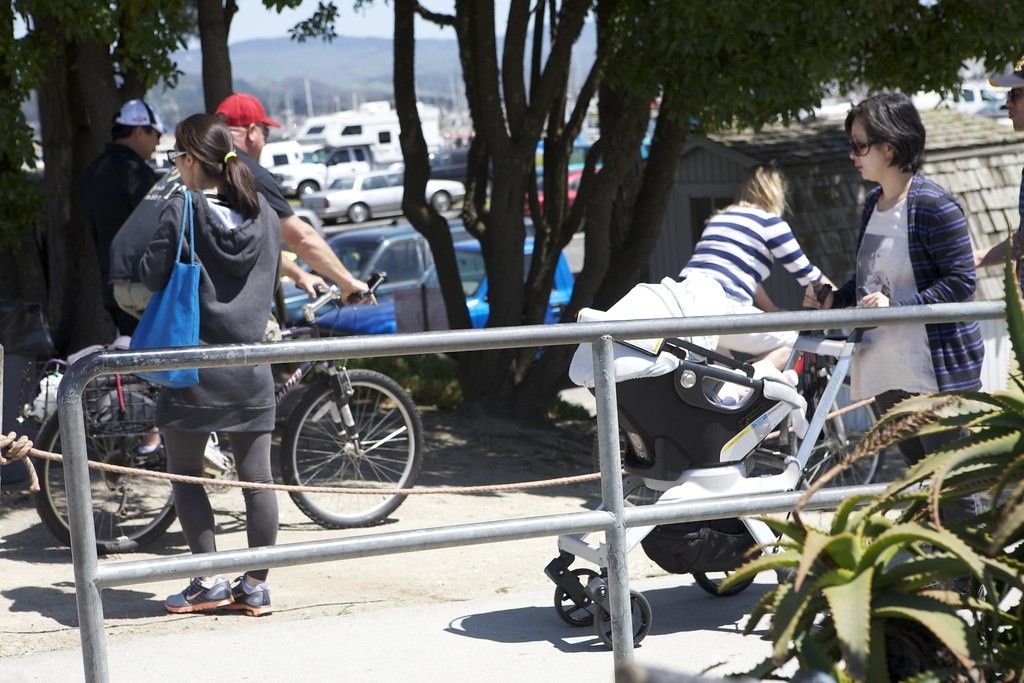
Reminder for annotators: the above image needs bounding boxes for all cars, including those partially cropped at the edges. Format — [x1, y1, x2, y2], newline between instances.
[145, 99, 700, 357]
[778, 56, 1013, 126]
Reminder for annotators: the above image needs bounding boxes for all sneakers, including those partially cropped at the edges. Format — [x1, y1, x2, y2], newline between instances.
[164, 577, 235, 613]
[222, 576, 272, 618]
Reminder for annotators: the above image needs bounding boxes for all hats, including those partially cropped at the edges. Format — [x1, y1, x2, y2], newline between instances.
[114, 97, 165, 132]
[989, 55, 1024, 87]
[215, 93, 281, 130]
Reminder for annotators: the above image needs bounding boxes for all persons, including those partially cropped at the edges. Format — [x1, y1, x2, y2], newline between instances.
[802, 92, 985, 599]
[138, 113, 281, 616]
[989, 54, 1024, 302]
[110, 92, 377, 471]
[82, 97, 175, 338]
[677, 164, 838, 372]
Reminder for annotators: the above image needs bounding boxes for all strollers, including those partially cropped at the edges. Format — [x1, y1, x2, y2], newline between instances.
[543, 277, 889, 651]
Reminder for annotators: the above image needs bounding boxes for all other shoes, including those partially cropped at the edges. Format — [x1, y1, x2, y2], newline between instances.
[130, 443, 168, 471]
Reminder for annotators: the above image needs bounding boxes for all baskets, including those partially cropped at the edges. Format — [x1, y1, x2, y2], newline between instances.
[25, 368, 65, 423]
[80, 374, 159, 439]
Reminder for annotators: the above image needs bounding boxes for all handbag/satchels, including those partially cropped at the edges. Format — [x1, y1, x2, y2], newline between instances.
[127, 191, 203, 387]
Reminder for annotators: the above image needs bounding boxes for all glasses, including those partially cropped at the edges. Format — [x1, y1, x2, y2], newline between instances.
[167, 149, 188, 164]
[849, 139, 878, 157]
[1006, 89, 1024, 101]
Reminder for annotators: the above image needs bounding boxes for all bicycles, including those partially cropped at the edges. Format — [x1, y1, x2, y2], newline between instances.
[591, 324, 888, 514]
[31, 271, 426, 555]
